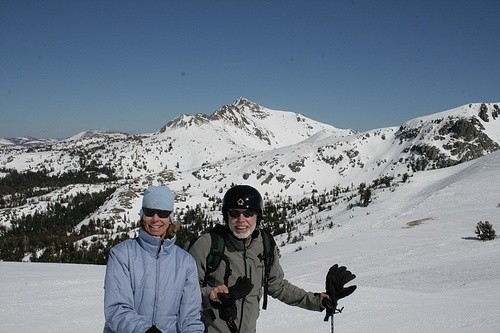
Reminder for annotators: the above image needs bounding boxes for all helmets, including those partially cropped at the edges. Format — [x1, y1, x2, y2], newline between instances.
[222, 185, 262, 216]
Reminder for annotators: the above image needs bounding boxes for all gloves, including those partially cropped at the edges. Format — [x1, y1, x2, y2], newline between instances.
[217, 276, 254, 306]
[322, 263, 357, 313]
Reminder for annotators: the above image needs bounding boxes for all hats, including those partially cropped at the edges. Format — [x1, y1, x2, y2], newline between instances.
[140, 186, 174, 223]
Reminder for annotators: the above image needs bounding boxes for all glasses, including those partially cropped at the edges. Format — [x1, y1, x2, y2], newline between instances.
[141, 208, 173, 218]
[228, 210, 256, 218]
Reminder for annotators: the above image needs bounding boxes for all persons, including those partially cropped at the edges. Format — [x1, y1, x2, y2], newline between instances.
[103, 185, 205, 333]
[188, 185, 332, 333]
